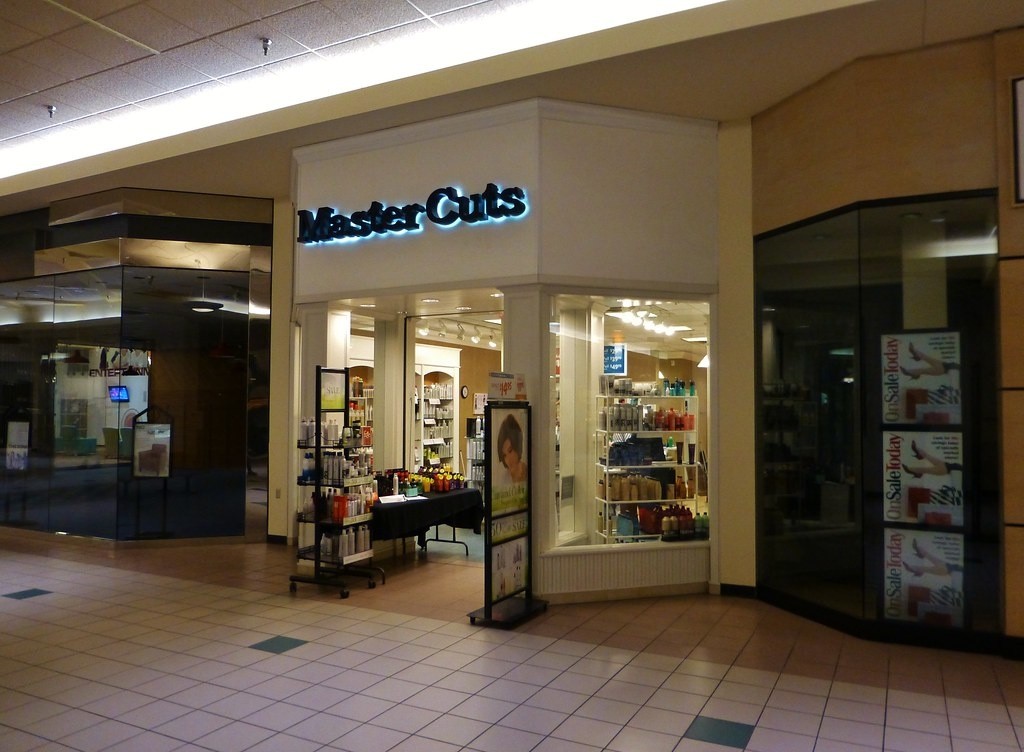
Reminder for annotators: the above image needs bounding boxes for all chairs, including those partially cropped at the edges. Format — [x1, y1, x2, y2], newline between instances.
[102, 427, 121, 459]
[119, 427, 133, 460]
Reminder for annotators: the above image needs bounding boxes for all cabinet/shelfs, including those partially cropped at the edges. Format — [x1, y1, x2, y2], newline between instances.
[464, 436, 485, 523]
[594, 394, 699, 545]
[289, 364, 376, 599]
[348, 335, 463, 473]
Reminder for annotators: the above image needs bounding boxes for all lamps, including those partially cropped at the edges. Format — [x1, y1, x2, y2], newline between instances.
[489, 329, 497, 347]
[456, 322, 465, 340]
[471, 325, 482, 343]
[438, 320, 448, 337]
[62, 349, 90, 363]
[418, 320, 430, 335]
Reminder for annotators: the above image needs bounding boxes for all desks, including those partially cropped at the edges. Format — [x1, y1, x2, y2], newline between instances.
[55, 438, 98, 456]
[337, 488, 484, 585]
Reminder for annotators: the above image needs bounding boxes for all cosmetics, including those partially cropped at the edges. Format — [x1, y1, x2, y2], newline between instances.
[300, 418, 374, 555]
[472, 415, 486, 503]
[348, 377, 373, 426]
[597, 375, 709, 544]
[375, 465, 464, 496]
[414, 382, 454, 471]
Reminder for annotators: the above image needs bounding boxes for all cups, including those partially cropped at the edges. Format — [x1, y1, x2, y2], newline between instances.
[666, 484, 675, 499]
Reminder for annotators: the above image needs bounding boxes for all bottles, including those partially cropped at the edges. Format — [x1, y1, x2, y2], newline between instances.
[658, 503, 709, 535]
[597, 471, 662, 501]
[599, 509, 640, 543]
[676, 476, 694, 498]
[384, 466, 465, 498]
[598, 402, 689, 431]
[301, 415, 361, 448]
[321, 483, 375, 524]
[475, 416, 481, 435]
[662, 377, 695, 396]
[302, 451, 346, 485]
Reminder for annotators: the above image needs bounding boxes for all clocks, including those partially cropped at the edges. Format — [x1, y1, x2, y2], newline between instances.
[461, 385, 469, 399]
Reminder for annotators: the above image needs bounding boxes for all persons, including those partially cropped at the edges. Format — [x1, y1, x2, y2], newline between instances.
[99, 347, 148, 369]
[902, 440, 962, 479]
[497, 415, 529, 484]
[900, 343, 960, 380]
[903, 538, 963, 577]
